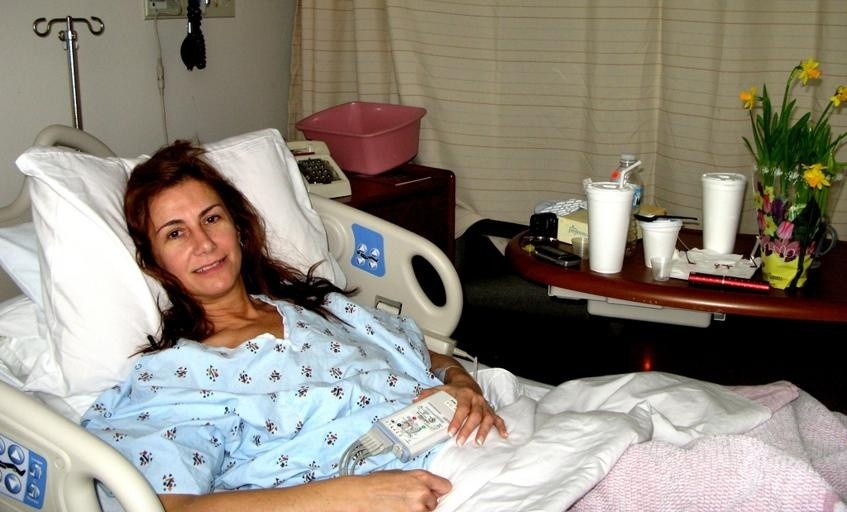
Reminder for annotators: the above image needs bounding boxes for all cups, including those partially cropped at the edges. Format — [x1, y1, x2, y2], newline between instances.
[586, 181, 636, 275]
[701, 173, 747, 255]
[650, 256, 672, 282]
[572, 237, 589, 260]
[640, 214, 683, 269]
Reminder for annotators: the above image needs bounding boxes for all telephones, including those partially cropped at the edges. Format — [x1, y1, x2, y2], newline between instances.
[287, 140, 352, 199]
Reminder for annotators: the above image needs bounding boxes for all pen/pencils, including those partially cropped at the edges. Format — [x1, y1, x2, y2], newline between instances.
[688, 272, 771, 293]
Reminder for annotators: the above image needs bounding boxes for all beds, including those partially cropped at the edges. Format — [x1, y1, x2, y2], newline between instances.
[0, 123, 847, 512]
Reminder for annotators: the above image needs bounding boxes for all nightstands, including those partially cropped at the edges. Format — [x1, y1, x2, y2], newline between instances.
[333, 161, 457, 269]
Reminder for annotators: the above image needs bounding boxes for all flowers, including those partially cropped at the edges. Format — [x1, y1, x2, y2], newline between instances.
[740, 58, 847, 260]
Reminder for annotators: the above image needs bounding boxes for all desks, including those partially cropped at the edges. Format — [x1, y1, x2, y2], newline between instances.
[504, 225, 847, 371]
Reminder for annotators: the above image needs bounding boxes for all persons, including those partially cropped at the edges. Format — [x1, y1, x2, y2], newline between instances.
[76, 137, 509, 512]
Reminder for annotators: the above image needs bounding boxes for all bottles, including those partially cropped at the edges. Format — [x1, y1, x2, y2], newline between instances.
[610, 152, 644, 258]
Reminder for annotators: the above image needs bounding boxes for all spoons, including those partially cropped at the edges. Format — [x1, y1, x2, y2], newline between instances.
[632, 213, 699, 223]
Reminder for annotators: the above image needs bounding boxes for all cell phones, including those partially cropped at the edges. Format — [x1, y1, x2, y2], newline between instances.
[534, 245, 582, 267]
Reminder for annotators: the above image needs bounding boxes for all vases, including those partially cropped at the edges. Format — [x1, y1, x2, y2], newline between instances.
[752, 162, 846, 290]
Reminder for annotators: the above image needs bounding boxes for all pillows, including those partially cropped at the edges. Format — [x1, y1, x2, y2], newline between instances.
[3, 294, 104, 424]
[15, 128, 346, 395]
[1, 224, 46, 308]
